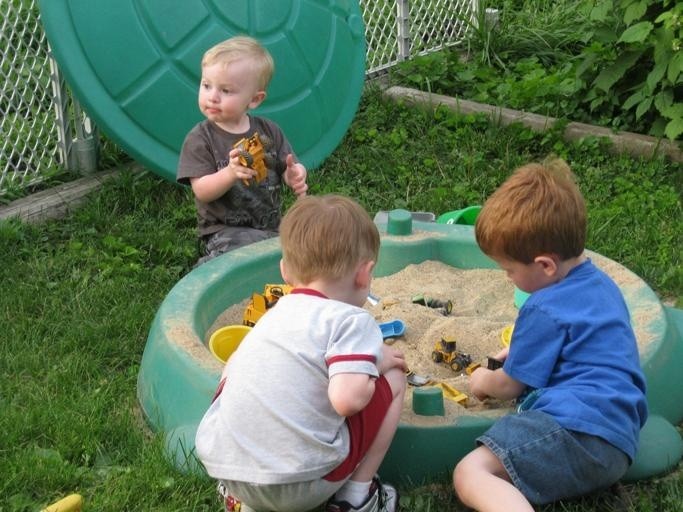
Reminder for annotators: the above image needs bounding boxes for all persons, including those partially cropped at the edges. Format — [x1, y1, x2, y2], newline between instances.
[452, 155, 648, 512]
[195, 194, 408, 512]
[176, 36, 309, 270]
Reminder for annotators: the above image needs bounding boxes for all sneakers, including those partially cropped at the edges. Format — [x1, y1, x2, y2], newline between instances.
[324, 473, 398, 512]
